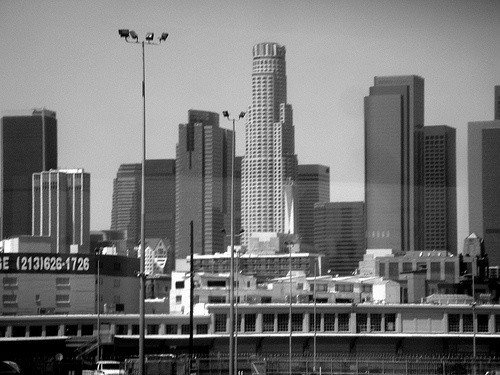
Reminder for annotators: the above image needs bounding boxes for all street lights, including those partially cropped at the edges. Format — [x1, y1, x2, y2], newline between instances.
[118, 28, 169, 375]
[222, 110, 246, 375]
[284, 239, 294, 375]
[92, 244, 104, 368]
